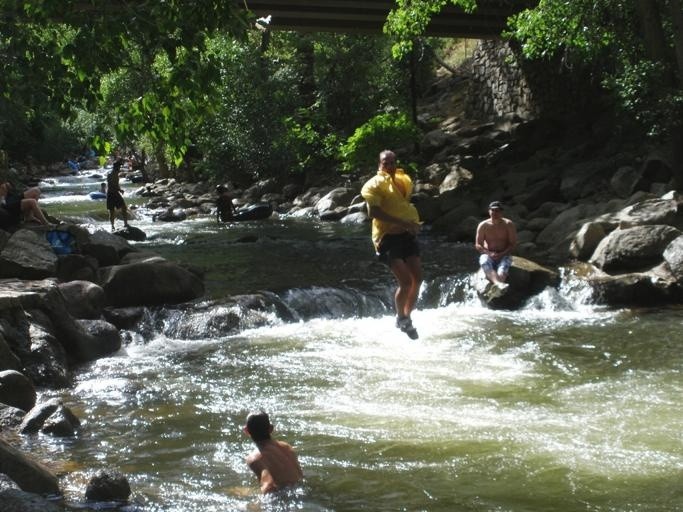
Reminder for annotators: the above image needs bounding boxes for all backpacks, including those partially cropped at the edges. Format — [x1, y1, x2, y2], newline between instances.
[46, 230, 78, 254]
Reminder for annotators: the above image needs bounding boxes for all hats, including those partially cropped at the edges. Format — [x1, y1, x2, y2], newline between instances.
[489, 201, 504, 210]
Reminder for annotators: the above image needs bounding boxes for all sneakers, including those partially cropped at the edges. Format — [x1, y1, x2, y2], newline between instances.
[396, 315, 418, 339]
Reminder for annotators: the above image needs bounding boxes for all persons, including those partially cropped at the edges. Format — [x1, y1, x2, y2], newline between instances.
[99, 183, 106, 194]
[106, 148, 136, 171]
[475, 201, 517, 291]
[243, 411, 303, 493]
[0, 182, 55, 225]
[215, 185, 238, 223]
[361, 150, 423, 340]
[107, 161, 130, 233]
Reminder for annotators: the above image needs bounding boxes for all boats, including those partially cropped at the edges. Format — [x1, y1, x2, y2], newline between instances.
[232, 202, 271, 220]
[89, 191, 106, 199]
